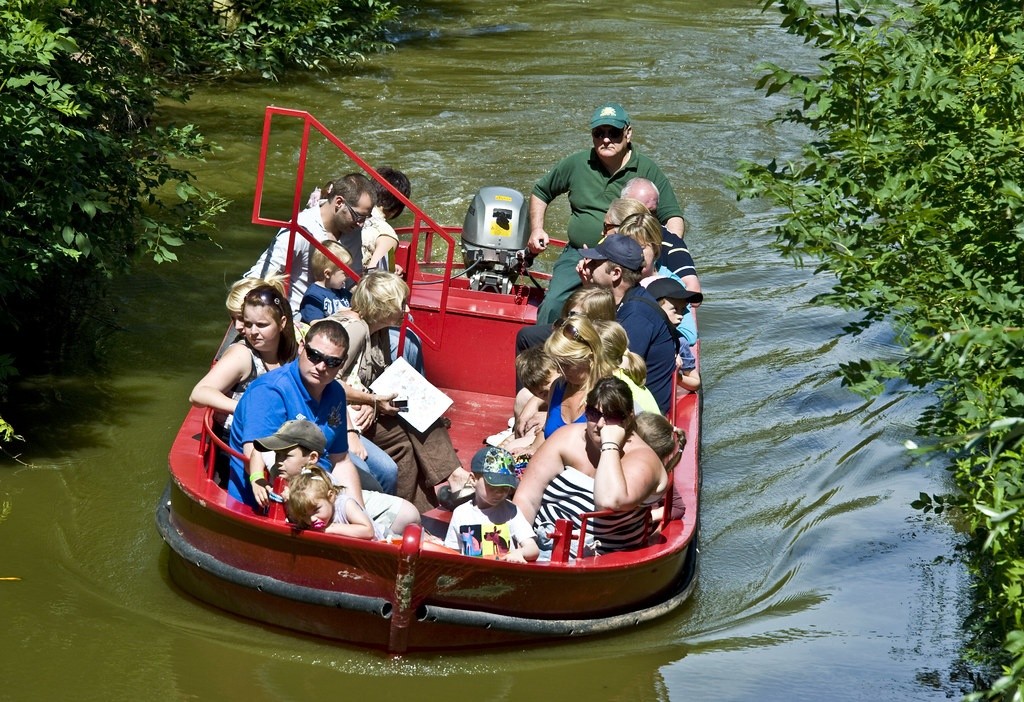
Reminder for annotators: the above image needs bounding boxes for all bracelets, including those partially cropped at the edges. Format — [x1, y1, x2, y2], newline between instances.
[347, 429, 360, 438]
[250, 471, 264, 482]
[600, 442, 621, 452]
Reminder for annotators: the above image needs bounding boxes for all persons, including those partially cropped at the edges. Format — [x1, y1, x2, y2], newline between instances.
[361, 167, 404, 281]
[309, 271, 477, 515]
[634, 411, 686, 523]
[244, 172, 451, 428]
[249, 420, 337, 508]
[512, 377, 668, 561]
[299, 240, 352, 325]
[227, 320, 421, 534]
[527, 101, 685, 323]
[486, 178, 704, 447]
[446, 445, 543, 563]
[288, 464, 401, 543]
[189, 276, 398, 494]
[514, 314, 612, 480]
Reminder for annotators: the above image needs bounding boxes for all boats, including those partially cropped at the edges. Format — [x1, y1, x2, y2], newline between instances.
[153, 105, 703, 654]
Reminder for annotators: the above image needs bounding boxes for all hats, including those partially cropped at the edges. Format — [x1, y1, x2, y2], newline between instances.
[578, 234, 644, 271]
[592, 103, 630, 130]
[471, 446, 517, 489]
[647, 277, 703, 304]
[253, 420, 326, 455]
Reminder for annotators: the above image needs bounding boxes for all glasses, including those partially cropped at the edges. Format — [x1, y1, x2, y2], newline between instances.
[592, 125, 629, 139]
[553, 319, 591, 349]
[304, 344, 345, 368]
[602, 222, 620, 233]
[243, 289, 284, 315]
[586, 407, 627, 425]
[338, 194, 370, 224]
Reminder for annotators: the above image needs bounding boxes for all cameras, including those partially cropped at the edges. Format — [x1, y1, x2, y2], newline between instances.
[389, 395, 408, 408]
